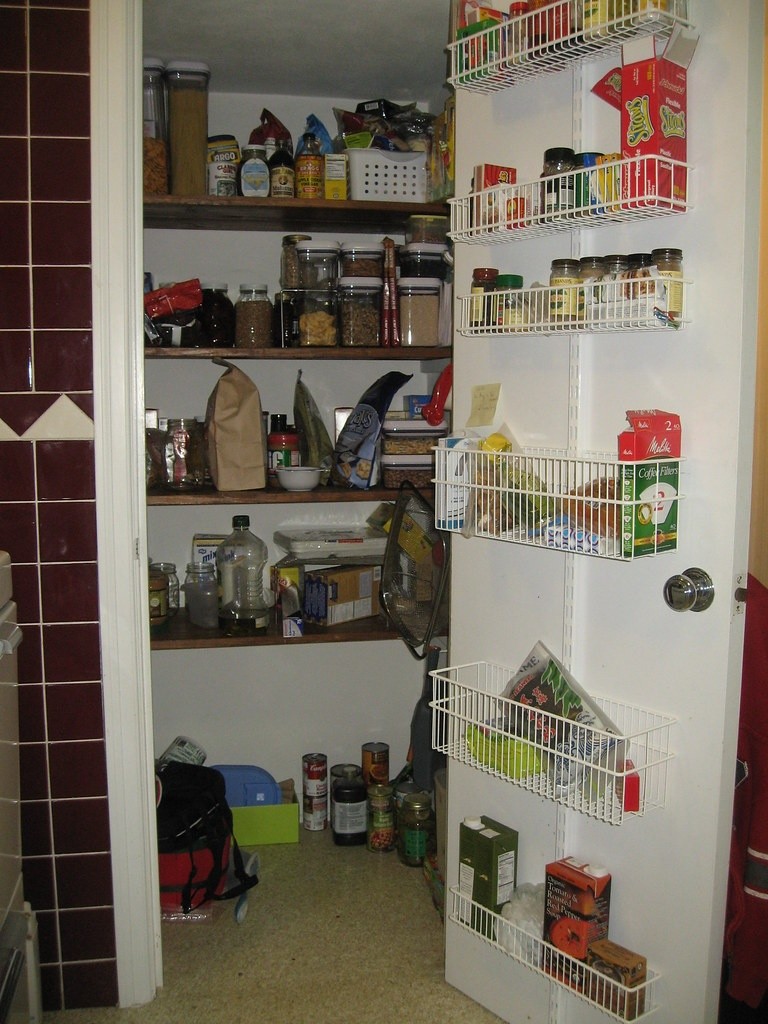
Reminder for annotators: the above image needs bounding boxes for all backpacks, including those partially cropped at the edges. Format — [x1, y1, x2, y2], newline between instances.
[158, 760, 259, 913]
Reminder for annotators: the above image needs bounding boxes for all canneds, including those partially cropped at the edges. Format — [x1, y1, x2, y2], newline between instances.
[201, 234, 314, 348]
[165, 415, 301, 491]
[539, 147, 607, 223]
[468, 248, 684, 329]
[207, 135, 271, 199]
[303, 743, 435, 867]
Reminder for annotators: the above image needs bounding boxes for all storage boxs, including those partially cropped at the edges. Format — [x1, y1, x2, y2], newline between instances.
[231, 790, 301, 847]
[587, 940, 646, 1020]
[617, 408, 682, 556]
[333, 395, 446, 486]
[424, 853, 446, 920]
[590, 23, 700, 213]
[455, 3, 511, 89]
[341, 148, 427, 202]
[192, 532, 244, 610]
[323, 154, 347, 200]
[270, 503, 432, 626]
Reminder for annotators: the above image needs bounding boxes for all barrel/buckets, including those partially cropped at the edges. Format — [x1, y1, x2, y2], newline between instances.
[208, 160, 238, 197]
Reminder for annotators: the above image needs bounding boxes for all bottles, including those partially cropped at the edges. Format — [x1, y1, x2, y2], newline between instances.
[207, 133, 240, 164]
[199, 235, 446, 351]
[540, 147, 576, 219]
[162, 417, 205, 490]
[331, 766, 369, 846]
[467, 267, 498, 334]
[508, 2, 528, 65]
[240, 133, 323, 198]
[155, 563, 181, 618]
[571, 0, 672, 46]
[184, 562, 215, 615]
[399, 793, 436, 867]
[268, 429, 300, 487]
[217, 515, 270, 636]
[149, 570, 169, 625]
[165, 60, 211, 196]
[496, 274, 523, 333]
[550, 248, 684, 328]
[141, 56, 168, 197]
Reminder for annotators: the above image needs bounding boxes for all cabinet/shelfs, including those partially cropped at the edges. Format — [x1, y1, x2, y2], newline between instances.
[144, 194, 450, 650]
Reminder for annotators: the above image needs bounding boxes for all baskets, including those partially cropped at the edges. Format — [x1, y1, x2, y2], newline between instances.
[342, 148, 427, 203]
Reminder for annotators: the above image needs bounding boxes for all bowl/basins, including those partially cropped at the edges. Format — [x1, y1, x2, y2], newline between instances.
[184, 582, 216, 629]
[381, 454, 435, 489]
[272, 467, 322, 491]
[380, 418, 448, 455]
[401, 216, 448, 244]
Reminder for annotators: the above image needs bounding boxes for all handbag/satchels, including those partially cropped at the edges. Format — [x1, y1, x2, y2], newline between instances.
[204, 357, 267, 491]
